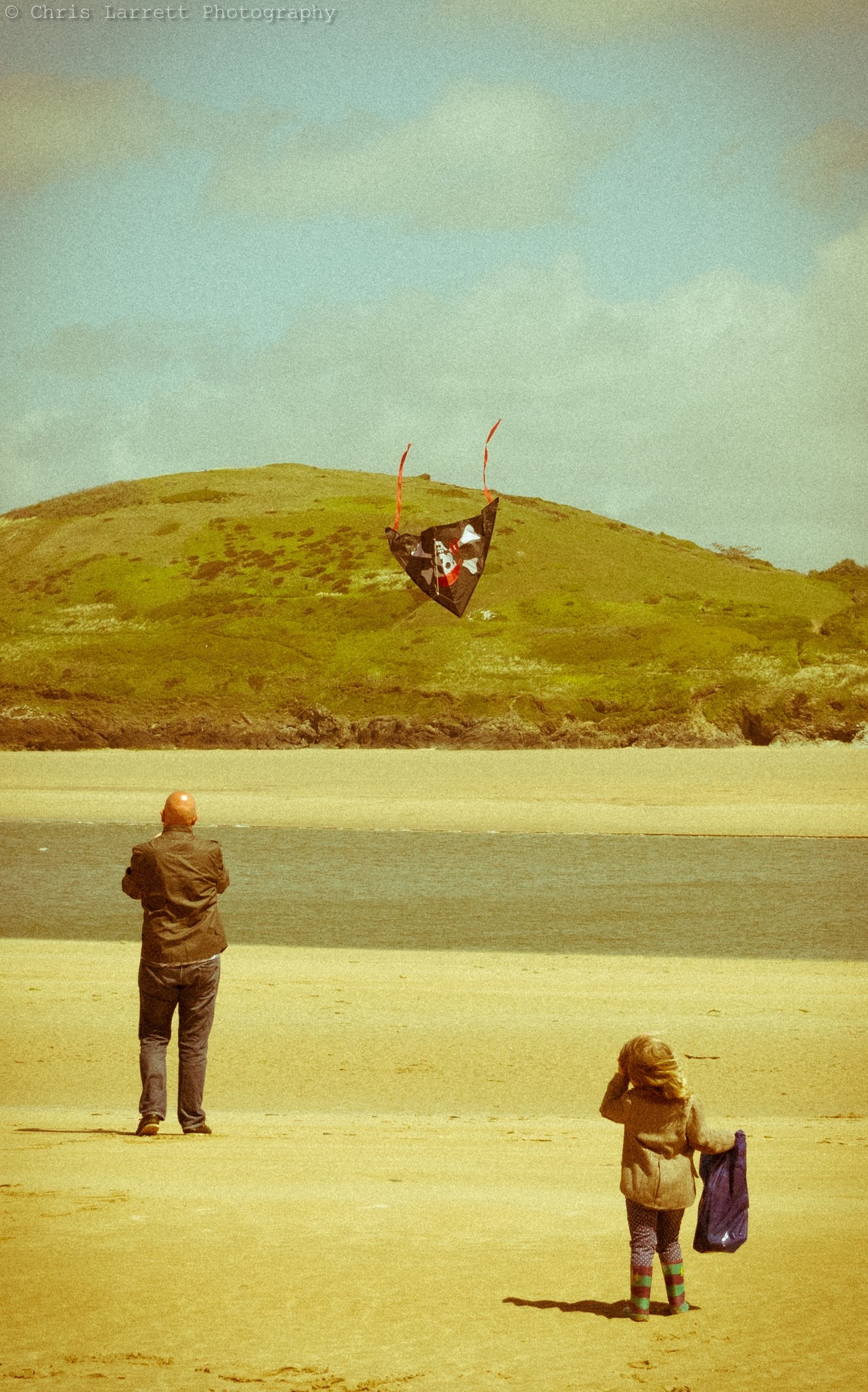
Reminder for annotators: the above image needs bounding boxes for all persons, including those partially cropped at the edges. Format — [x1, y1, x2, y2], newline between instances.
[599, 1036, 733, 1320]
[122, 793, 229, 1133]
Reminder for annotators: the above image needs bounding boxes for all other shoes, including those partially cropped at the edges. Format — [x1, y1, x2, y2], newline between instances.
[183, 1124, 212, 1134]
[135, 1114, 160, 1135]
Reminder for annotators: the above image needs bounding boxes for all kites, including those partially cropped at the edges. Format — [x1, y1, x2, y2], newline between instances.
[386, 419, 499, 618]
